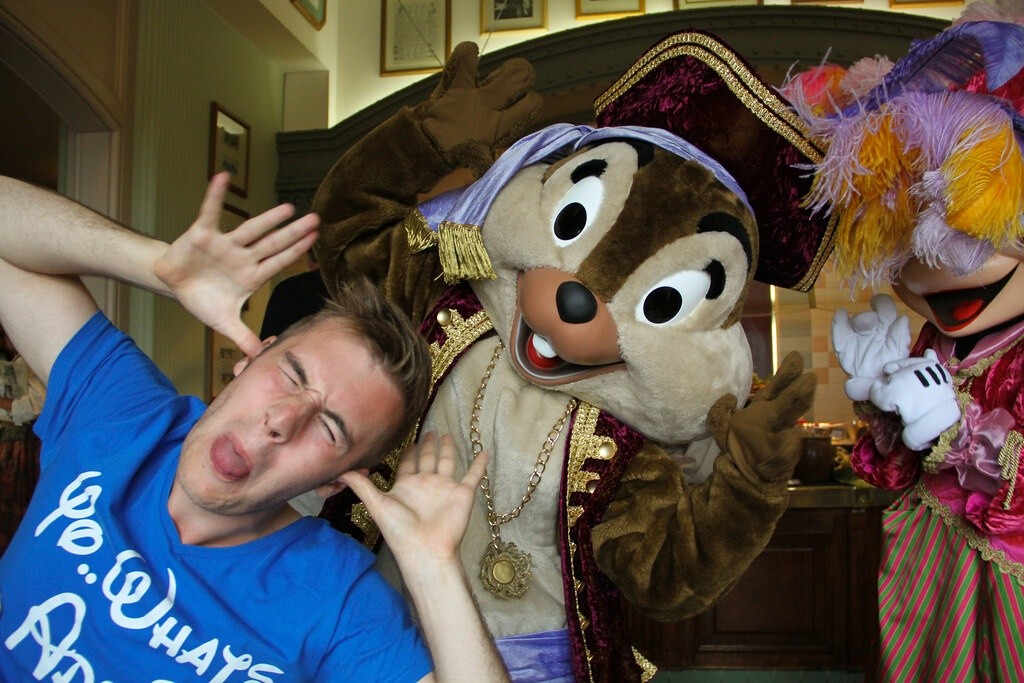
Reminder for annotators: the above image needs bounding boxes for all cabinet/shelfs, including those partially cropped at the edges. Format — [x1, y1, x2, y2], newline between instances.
[630, 510, 881, 673]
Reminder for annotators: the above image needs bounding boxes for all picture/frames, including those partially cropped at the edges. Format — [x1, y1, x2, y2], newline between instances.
[888, 0, 966, 11]
[574, 0, 646, 21]
[479, 0, 548, 37]
[672, 0, 764, 10]
[290, 0, 327, 31]
[207, 100, 251, 198]
[380, 0, 452, 77]
[219, 202, 250, 233]
[204, 326, 248, 405]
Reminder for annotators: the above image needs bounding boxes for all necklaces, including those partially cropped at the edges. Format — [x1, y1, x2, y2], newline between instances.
[469, 338, 576, 601]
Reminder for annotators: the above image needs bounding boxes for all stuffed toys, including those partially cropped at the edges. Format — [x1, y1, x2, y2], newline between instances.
[771, 0, 1024, 683]
[311, 29, 846, 683]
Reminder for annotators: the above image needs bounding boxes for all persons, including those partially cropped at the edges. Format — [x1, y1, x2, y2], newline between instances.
[258, 244, 333, 341]
[0, 325, 47, 561]
[0, 172, 513, 683]
